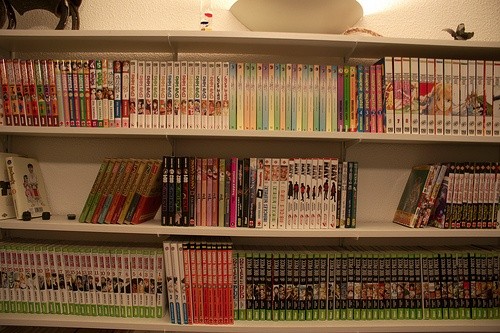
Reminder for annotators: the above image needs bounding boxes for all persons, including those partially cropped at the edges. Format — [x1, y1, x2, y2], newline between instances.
[23, 163, 45, 207]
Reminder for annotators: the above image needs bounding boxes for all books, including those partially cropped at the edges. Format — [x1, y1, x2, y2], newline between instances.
[0, 152, 19, 220]
[1, 58, 229, 131]
[78, 157, 163, 225]
[0, 238, 168, 319]
[393, 164, 446, 228]
[372, 57, 500, 138]
[162, 236, 234, 326]
[162, 154, 359, 230]
[233, 244, 500, 323]
[229, 62, 384, 134]
[5, 157, 52, 220]
[428, 162, 500, 229]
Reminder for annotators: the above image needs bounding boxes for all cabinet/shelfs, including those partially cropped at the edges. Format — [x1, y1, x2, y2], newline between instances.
[0, 29, 500, 333]
